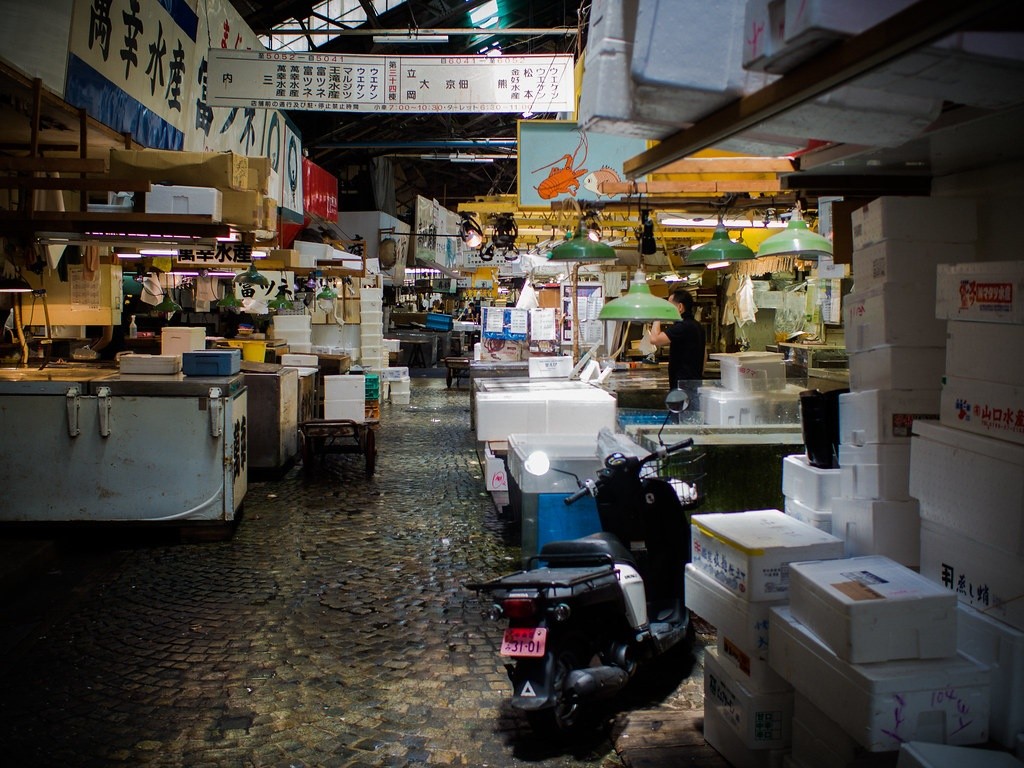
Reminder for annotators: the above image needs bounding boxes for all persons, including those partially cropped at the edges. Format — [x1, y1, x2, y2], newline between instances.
[432, 299, 444, 314]
[91, 324, 125, 360]
[649, 289, 706, 410]
[456, 300, 480, 323]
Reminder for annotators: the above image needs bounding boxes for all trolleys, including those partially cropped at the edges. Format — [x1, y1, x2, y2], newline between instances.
[443, 356, 474, 388]
[299, 418, 380, 477]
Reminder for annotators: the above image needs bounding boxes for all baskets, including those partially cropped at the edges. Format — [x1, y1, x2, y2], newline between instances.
[277, 301, 304, 315]
[631, 433, 710, 510]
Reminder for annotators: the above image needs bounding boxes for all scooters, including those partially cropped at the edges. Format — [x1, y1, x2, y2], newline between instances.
[464, 388, 708, 750]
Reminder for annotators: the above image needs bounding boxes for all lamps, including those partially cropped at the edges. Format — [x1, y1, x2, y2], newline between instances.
[457, 210, 483, 248]
[597, 239, 684, 321]
[633, 210, 658, 255]
[686, 210, 756, 270]
[547, 219, 619, 262]
[582, 211, 601, 242]
[755, 209, 833, 258]
[0, 253, 34, 292]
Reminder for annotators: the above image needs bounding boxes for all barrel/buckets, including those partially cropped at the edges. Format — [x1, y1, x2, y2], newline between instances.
[631, 340, 641, 349]
[243, 342, 268, 363]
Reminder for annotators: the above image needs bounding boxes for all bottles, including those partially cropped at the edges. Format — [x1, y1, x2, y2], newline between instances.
[471, 295, 474, 302]
[476, 290, 480, 300]
[458, 308, 462, 315]
[465, 294, 468, 299]
[130, 315, 137, 338]
[294, 272, 338, 310]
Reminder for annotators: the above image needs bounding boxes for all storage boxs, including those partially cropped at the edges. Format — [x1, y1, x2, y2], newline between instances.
[119, 240, 411, 425]
[425, 308, 663, 575]
[109, 147, 279, 230]
[684, 196, 1024, 768]
[552, 0, 1024, 149]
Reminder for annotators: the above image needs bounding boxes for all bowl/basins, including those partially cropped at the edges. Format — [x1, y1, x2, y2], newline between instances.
[238, 324, 254, 334]
